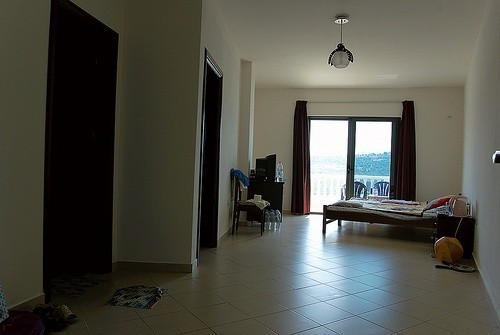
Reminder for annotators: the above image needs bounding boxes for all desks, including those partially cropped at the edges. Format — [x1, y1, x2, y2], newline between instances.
[247, 182, 285, 224]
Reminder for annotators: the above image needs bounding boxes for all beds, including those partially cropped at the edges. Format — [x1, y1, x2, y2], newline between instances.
[323, 198, 470, 235]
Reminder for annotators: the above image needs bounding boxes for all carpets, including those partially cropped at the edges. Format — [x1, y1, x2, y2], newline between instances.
[104, 284, 169, 309]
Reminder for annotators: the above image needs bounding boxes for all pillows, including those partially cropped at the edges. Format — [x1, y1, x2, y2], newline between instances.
[333, 200, 363, 208]
[423, 197, 452, 213]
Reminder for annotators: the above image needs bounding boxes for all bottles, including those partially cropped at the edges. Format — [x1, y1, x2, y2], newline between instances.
[249, 170, 256, 184]
[264, 212, 270, 231]
[433, 221, 437, 233]
[269, 210, 276, 231]
[275, 210, 281, 230]
[277, 161, 283, 182]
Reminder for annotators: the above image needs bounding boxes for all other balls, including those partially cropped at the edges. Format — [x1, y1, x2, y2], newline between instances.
[434, 236, 464, 265]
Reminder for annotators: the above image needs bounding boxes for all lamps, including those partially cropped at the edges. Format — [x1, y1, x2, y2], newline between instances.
[328, 18, 353, 69]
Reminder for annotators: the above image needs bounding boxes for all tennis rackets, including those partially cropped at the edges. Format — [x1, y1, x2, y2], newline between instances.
[435, 263, 476, 273]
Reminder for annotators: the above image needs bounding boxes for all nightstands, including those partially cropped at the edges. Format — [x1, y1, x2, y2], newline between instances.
[431, 211, 476, 263]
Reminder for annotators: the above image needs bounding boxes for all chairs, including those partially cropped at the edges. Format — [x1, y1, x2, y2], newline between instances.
[230, 168, 270, 237]
[340, 181, 390, 201]
